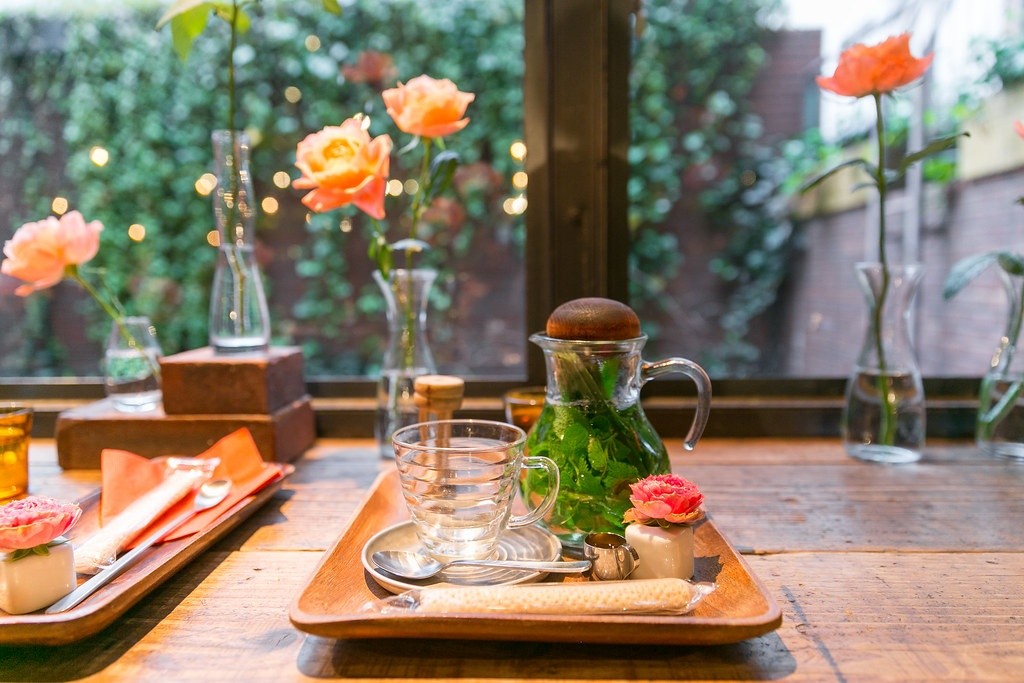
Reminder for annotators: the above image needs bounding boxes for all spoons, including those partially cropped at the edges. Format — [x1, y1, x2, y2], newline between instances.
[372, 551, 592, 579]
[46, 480, 232, 611]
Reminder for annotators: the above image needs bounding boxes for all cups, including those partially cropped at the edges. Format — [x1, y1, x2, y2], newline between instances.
[583, 531, 639, 582]
[507, 387, 549, 435]
[0, 407, 33, 503]
[391, 419, 559, 559]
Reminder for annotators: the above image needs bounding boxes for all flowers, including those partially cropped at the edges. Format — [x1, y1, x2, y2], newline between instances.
[797, 31, 969, 447]
[621, 474, 706, 529]
[0, 210, 161, 387]
[292, 73, 475, 443]
[942, 117, 1024, 444]
[0, 497, 83, 560]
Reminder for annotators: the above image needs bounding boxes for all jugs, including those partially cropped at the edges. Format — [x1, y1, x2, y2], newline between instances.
[523, 298, 712, 547]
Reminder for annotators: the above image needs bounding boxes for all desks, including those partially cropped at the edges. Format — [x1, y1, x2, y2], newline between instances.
[0, 437, 1023, 683]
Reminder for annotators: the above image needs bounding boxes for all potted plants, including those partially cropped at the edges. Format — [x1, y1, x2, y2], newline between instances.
[153, 0, 343, 354]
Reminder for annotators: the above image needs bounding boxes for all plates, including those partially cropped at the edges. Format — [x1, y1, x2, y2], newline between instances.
[362, 521, 561, 596]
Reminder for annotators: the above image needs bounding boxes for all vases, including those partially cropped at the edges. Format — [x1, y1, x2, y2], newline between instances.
[103, 317, 163, 413]
[371, 269, 438, 459]
[974, 268, 1024, 465]
[843, 262, 928, 462]
